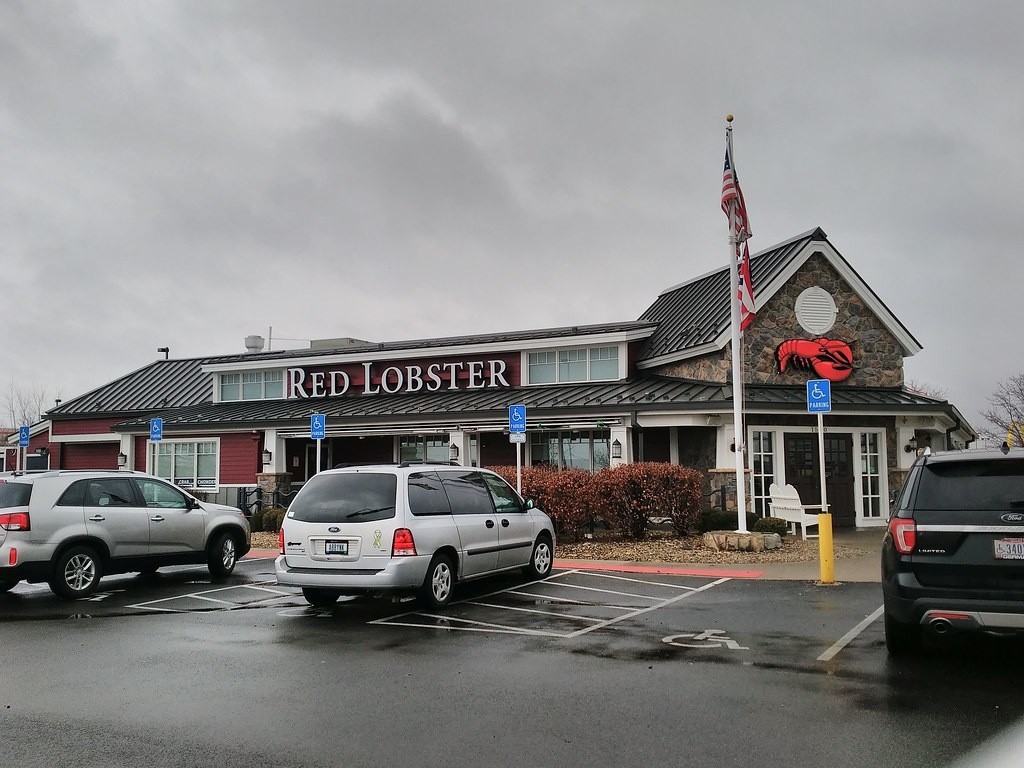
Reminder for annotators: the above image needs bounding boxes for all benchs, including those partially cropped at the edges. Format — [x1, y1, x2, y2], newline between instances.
[767, 483, 831, 540]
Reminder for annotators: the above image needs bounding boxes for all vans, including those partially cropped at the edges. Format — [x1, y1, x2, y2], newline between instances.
[273, 462, 559, 612]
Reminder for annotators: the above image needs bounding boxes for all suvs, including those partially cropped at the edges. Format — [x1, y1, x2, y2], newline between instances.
[880, 445, 1024, 662]
[0, 463, 252, 604]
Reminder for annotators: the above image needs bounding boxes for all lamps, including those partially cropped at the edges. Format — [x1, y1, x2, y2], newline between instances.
[117, 452, 127, 466]
[449, 443, 459, 460]
[904, 436, 918, 453]
[261, 449, 272, 464]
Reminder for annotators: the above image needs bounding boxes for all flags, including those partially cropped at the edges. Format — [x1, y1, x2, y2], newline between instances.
[737, 244, 756, 329]
[720, 133, 756, 242]
